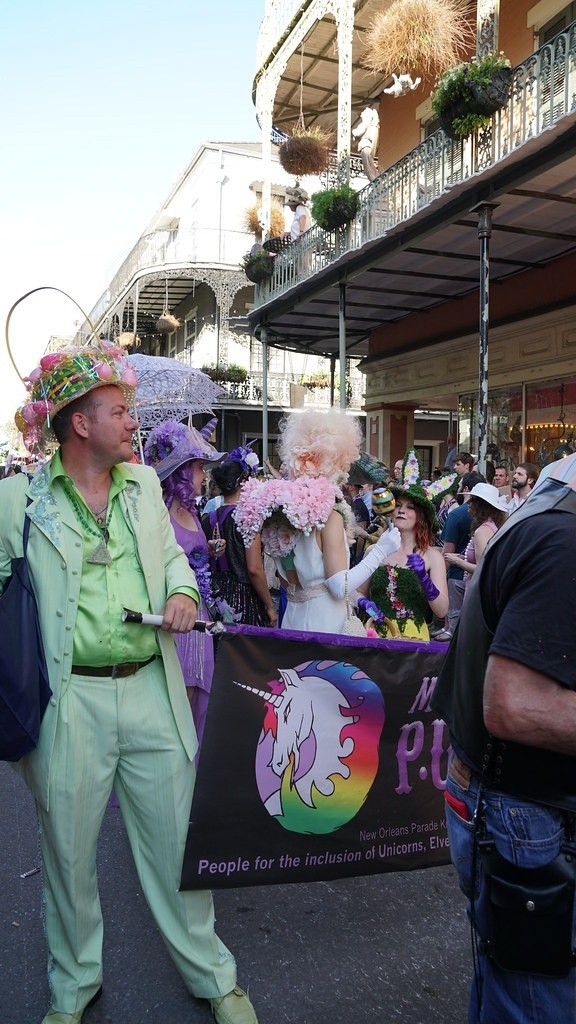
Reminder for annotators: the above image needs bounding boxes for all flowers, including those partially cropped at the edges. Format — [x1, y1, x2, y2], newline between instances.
[400, 450, 457, 500]
[386, 562, 416, 619]
[245, 452, 260, 467]
[232, 476, 344, 558]
[188, 545, 209, 568]
[289, 449, 350, 487]
[144, 416, 185, 467]
[429, 48, 510, 120]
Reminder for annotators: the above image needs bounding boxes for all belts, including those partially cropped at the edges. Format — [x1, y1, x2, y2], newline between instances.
[72, 655, 166, 680]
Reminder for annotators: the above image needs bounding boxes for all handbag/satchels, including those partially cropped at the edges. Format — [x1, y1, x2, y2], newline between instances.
[0, 474, 52, 765]
[477, 841, 576, 977]
[343, 616, 367, 637]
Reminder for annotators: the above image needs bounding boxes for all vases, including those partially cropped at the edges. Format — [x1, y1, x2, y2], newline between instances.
[310, 178, 359, 232]
[437, 66, 509, 141]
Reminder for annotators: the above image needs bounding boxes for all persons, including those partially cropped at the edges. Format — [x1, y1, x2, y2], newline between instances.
[431, 479, 576, 1024]
[1, 449, 26, 477]
[0, 346, 260, 1024]
[234, 404, 405, 638]
[140, 417, 223, 760]
[199, 438, 575, 584]
[348, 482, 448, 641]
[431, 482, 510, 641]
[202, 443, 277, 634]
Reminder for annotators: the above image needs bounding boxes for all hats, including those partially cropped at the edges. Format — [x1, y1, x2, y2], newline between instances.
[12, 344, 136, 456]
[387, 446, 457, 532]
[143, 414, 227, 486]
[457, 483, 509, 513]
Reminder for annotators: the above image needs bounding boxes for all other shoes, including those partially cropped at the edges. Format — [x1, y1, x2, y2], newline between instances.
[40, 984, 103, 1024]
[206, 983, 259, 1024]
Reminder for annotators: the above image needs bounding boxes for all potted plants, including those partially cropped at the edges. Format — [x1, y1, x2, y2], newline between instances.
[239, 250, 274, 283]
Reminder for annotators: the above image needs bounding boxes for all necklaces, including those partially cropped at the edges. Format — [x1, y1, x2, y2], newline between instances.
[462, 535, 474, 581]
[92, 507, 108, 524]
[57, 479, 115, 564]
